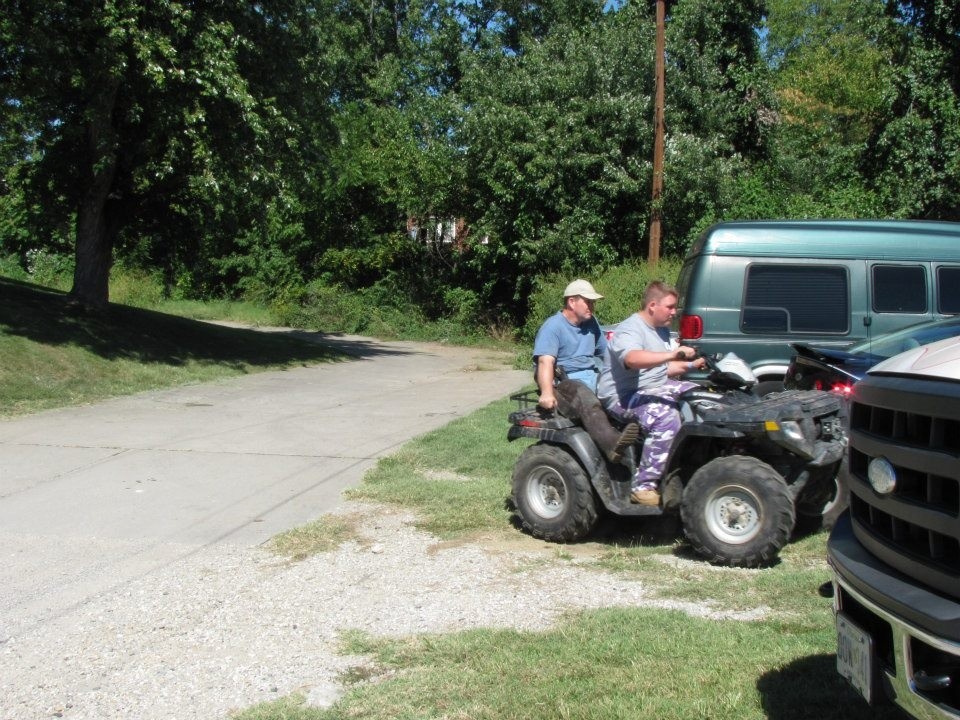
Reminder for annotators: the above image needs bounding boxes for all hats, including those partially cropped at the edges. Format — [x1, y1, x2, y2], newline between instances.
[564, 279, 606, 301]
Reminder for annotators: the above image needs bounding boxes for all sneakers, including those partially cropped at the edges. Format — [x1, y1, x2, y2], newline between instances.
[630, 490, 660, 505]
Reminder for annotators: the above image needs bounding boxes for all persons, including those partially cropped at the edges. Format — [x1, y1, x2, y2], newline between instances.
[597, 282, 708, 505]
[533, 279, 640, 465]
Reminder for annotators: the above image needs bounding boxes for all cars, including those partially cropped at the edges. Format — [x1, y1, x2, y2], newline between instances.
[782, 314, 960, 401]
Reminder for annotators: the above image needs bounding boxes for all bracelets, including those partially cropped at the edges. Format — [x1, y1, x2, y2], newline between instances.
[688, 361, 693, 371]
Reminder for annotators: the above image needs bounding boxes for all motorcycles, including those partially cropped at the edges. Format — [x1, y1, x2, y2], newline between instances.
[507, 346, 850, 569]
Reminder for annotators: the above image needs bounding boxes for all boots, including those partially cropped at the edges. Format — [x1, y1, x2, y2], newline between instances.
[585, 411, 639, 464]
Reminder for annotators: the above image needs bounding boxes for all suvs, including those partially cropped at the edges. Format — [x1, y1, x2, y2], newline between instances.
[818, 334, 960, 720]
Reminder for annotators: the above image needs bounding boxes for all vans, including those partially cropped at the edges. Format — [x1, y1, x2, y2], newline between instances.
[666, 220, 960, 394]
